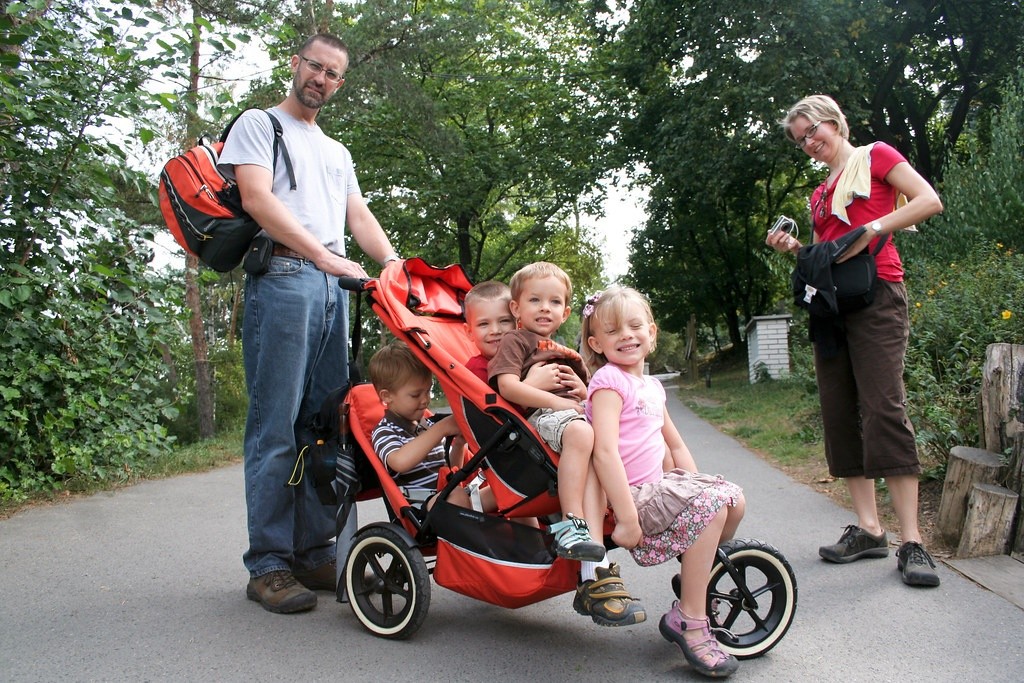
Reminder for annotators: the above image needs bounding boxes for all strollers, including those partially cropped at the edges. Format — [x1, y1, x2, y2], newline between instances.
[338, 257, 798, 660]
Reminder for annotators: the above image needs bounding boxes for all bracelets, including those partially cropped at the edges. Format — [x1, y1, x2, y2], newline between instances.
[383, 255, 402, 268]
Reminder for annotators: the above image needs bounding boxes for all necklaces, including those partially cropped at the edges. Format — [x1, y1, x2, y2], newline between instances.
[818, 168, 838, 217]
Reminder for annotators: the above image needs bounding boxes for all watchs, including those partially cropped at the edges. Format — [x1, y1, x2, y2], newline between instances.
[871, 220, 883, 236]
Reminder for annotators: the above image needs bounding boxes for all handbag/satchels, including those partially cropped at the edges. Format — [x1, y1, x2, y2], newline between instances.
[286, 381, 379, 500]
[790, 224, 890, 317]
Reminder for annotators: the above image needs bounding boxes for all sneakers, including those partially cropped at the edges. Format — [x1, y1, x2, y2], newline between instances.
[546, 512, 606, 562]
[659, 605, 738, 677]
[572, 564, 647, 628]
[672, 574, 724, 629]
[818, 524, 889, 563]
[898, 540, 939, 586]
[294, 559, 380, 595]
[247, 570, 317, 612]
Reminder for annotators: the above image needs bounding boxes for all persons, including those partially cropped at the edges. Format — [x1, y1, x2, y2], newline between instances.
[464, 262, 647, 627]
[368, 341, 552, 564]
[765, 94, 946, 589]
[580, 281, 753, 676]
[217, 33, 406, 614]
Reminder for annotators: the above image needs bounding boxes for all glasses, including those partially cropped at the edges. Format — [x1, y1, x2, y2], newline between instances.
[299, 54, 344, 83]
[794, 120, 821, 149]
[819, 188, 828, 220]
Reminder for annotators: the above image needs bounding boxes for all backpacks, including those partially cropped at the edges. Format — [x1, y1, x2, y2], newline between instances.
[158, 107, 296, 273]
